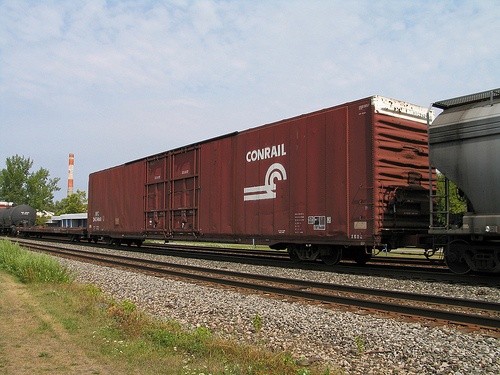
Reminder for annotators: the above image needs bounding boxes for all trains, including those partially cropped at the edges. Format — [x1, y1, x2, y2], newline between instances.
[0, 87, 500, 275]
